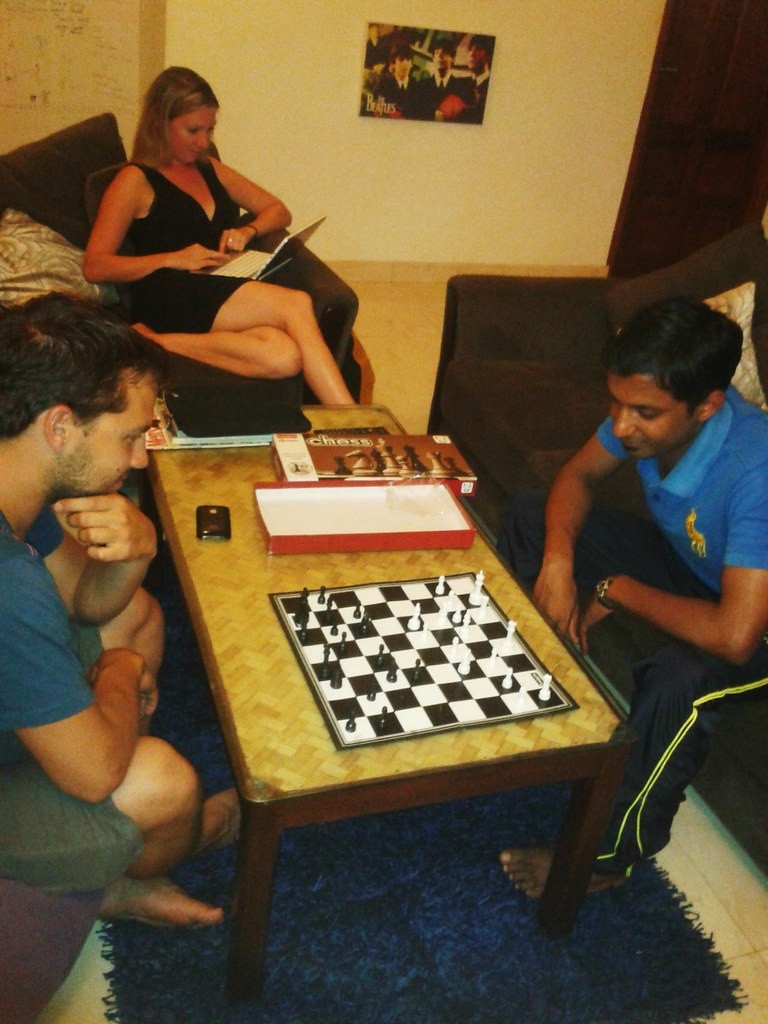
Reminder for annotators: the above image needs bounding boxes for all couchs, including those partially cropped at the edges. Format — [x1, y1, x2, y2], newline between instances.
[0, 211, 359, 409]
[425, 272, 768, 878]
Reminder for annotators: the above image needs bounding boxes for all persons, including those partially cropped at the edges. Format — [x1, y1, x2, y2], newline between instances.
[501, 295, 768, 897]
[82, 66, 357, 406]
[0, 292, 244, 933]
[370, 34, 495, 123]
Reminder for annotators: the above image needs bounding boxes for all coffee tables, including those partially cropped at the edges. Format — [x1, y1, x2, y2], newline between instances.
[149, 401, 636, 1006]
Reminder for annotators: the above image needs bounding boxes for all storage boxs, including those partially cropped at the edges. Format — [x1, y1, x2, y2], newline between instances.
[251, 480, 479, 556]
[270, 432, 477, 498]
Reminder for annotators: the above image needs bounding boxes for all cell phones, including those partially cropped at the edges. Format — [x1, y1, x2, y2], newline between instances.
[196, 505, 231, 539]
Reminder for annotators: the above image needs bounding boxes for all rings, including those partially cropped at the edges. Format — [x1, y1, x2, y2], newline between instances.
[226, 237, 233, 241]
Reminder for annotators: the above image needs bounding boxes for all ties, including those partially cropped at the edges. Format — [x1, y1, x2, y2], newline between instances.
[473, 77, 477, 87]
[400, 82, 405, 89]
[439, 80, 443, 87]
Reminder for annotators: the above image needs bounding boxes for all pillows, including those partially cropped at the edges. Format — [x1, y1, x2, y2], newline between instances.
[0, 206, 121, 309]
[614, 281, 766, 411]
[0, 111, 128, 249]
[603, 220, 768, 339]
[84, 140, 221, 227]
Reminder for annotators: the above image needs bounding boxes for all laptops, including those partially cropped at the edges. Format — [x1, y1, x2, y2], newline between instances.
[189, 214, 327, 281]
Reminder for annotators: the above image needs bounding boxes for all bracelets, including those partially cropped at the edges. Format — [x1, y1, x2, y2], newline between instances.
[244, 223, 258, 239]
[594, 576, 620, 610]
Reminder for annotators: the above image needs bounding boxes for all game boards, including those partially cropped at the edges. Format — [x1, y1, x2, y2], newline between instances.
[269, 570, 582, 750]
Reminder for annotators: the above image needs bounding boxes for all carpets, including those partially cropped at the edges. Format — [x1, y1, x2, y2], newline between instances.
[99, 575, 744, 1024]
